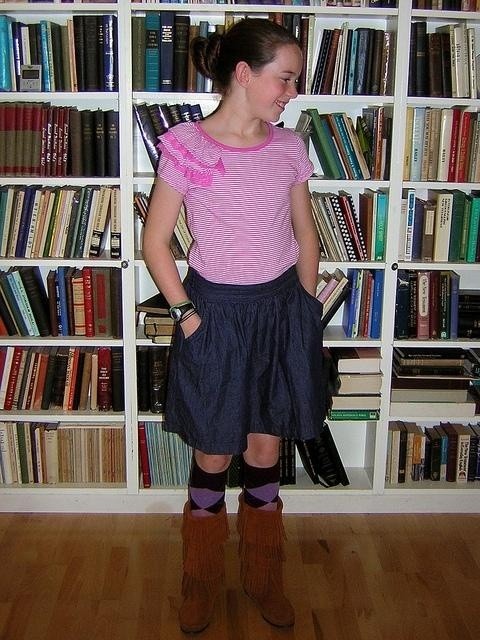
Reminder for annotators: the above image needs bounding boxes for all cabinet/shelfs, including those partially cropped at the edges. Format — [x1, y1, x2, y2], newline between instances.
[0, 2, 480, 517]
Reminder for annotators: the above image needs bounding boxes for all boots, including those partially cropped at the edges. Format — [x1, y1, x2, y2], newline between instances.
[237, 490, 296, 626]
[179, 500, 230, 631]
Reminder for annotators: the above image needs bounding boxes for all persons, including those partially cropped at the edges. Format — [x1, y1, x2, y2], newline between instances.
[143, 19, 333, 634]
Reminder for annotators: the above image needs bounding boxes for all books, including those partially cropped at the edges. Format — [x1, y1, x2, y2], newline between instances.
[1, 420, 126, 486]
[316, 269, 387, 421]
[403, 1, 479, 183]
[138, 420, 351, 488]
[385, 187, 479, 487]
[130, 1, 397, 95]
[134, 291, 180, 414]
[133, 101, 204, 260]
[2, 264, 125, 412]
[1, 1, 120, 93]
[1, 100, 122, 258]
[274, 103, 388, 262]
